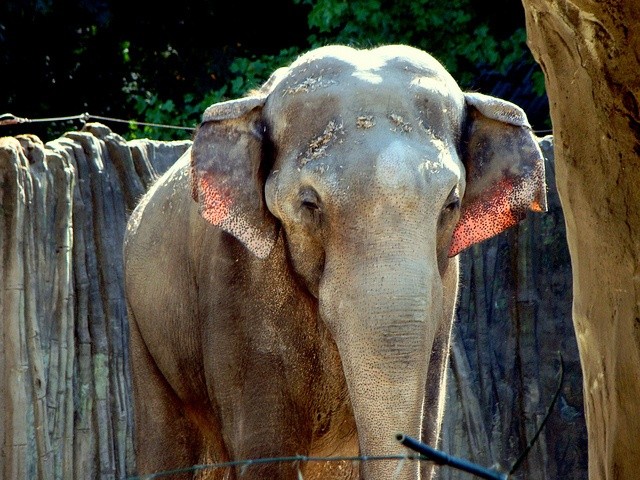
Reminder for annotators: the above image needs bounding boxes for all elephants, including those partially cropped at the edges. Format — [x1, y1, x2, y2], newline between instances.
[124, 43, 549, 480]
